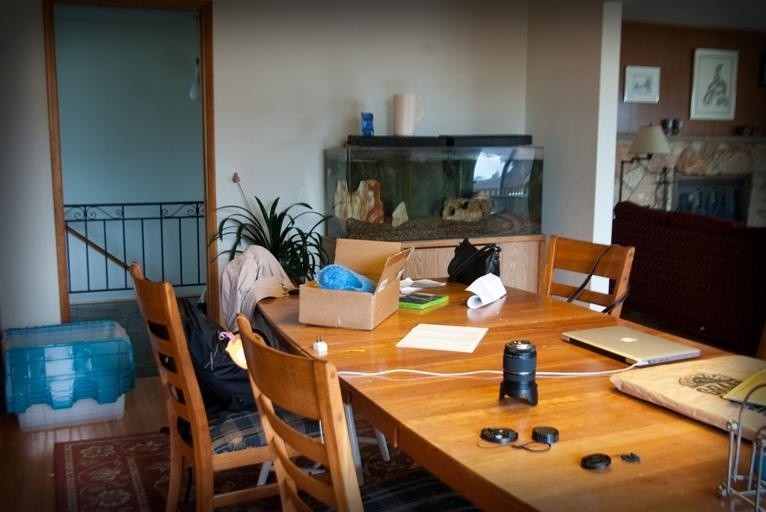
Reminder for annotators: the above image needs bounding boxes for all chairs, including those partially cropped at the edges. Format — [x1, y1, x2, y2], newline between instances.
[537, 235, 636, 318]
[129, 261, 333, 512]
[256, 312, 391, 487]
[235, 313, 482, 512]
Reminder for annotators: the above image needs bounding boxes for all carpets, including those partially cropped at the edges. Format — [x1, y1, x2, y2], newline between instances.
[53, 414, 485, 512]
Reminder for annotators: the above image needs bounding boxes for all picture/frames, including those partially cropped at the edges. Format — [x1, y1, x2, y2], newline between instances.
[689, 48, 739, 122]
[623, 65, 661, 104]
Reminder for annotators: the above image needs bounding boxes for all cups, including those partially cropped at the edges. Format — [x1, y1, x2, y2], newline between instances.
[673, 120, 684, 134]
[393, 90, 428, 138]
[661, 119, 672, 134]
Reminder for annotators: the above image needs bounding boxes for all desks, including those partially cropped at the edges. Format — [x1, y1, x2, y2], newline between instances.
[256, 277, 766, 512]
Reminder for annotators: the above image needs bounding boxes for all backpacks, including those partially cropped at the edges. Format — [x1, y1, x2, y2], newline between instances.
[148, 298, 269, 412]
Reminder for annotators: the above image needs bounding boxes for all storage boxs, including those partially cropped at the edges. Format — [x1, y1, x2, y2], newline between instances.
[298, 238, 415, 331]
[2, 319, 136, 433]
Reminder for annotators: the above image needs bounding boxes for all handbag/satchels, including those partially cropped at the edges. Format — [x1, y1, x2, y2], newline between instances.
[447, 239, 500, 284]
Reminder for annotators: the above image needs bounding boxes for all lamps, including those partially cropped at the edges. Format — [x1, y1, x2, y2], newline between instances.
[619, 122, 674, 202]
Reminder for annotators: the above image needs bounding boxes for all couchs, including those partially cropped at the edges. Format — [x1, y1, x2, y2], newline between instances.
[609, 201, 766, 359]
